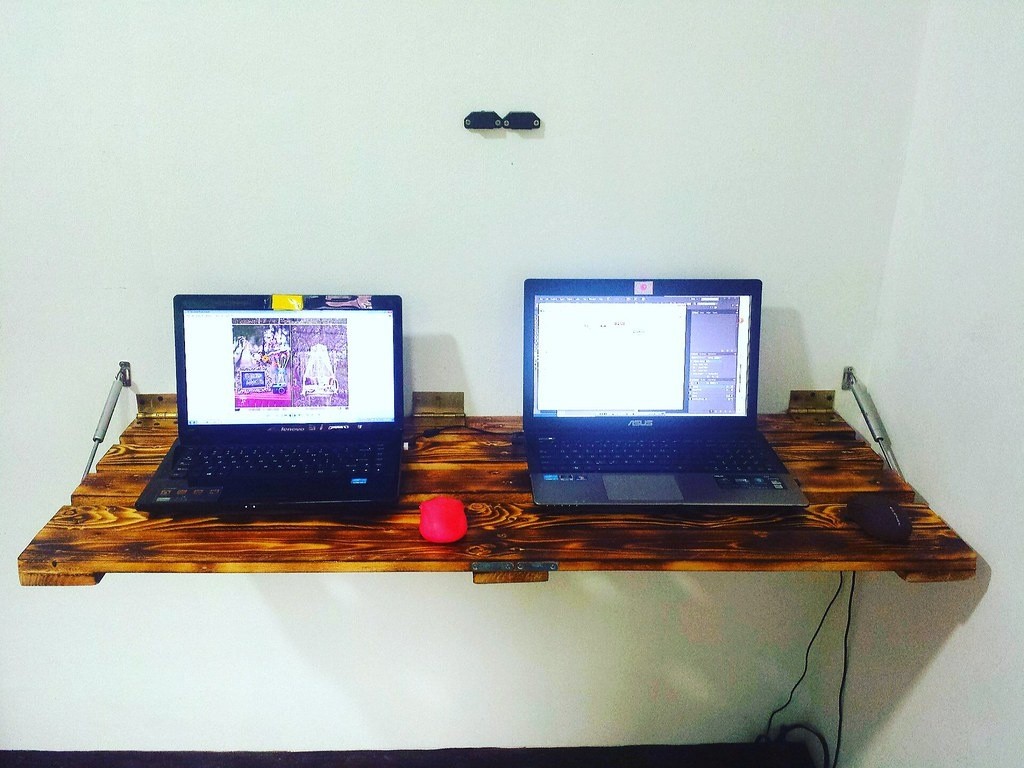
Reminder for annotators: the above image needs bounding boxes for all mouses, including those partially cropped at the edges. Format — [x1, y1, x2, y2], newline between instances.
[418, 497, 468, 544]
[842, 494, 914, 544]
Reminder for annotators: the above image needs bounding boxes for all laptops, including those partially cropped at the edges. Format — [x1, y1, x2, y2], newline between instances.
[524, 277, 811, 512]
[134, 294, 408, 511]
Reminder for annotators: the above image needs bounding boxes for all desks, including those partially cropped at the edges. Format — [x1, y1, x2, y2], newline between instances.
[18, 361, 977, 587]
